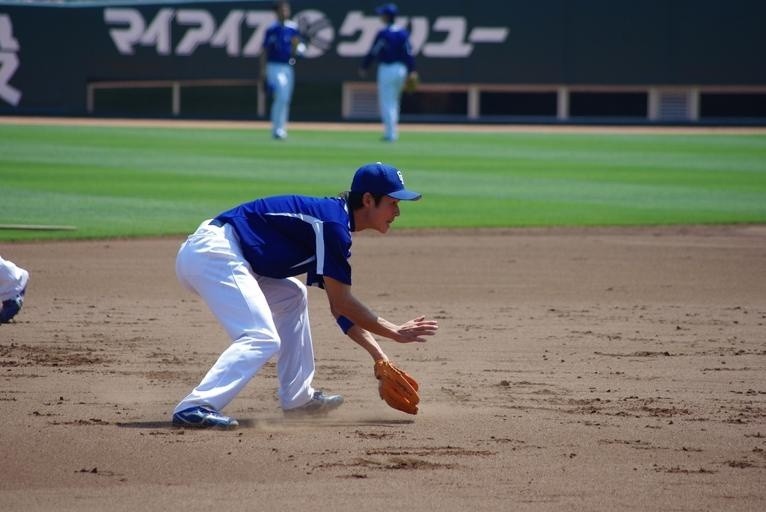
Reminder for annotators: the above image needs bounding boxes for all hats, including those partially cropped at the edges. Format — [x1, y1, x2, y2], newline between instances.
[374, 2, 398, 14]
[350, 162, 422, 201]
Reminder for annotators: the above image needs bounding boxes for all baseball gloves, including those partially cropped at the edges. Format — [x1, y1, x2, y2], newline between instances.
[374, 360, 421, 416]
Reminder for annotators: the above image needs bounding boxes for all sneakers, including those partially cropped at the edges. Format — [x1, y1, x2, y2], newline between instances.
[0, 289, 25, 322]
[172, 406, 239, 431]
[283, 390, 344, 417]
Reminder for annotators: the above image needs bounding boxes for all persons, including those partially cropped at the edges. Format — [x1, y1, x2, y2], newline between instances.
[168, 162, 441, 431]
[260, 1, 301, 142]
[358, 3, 420, 142]
[0, 255, 29, 325]
